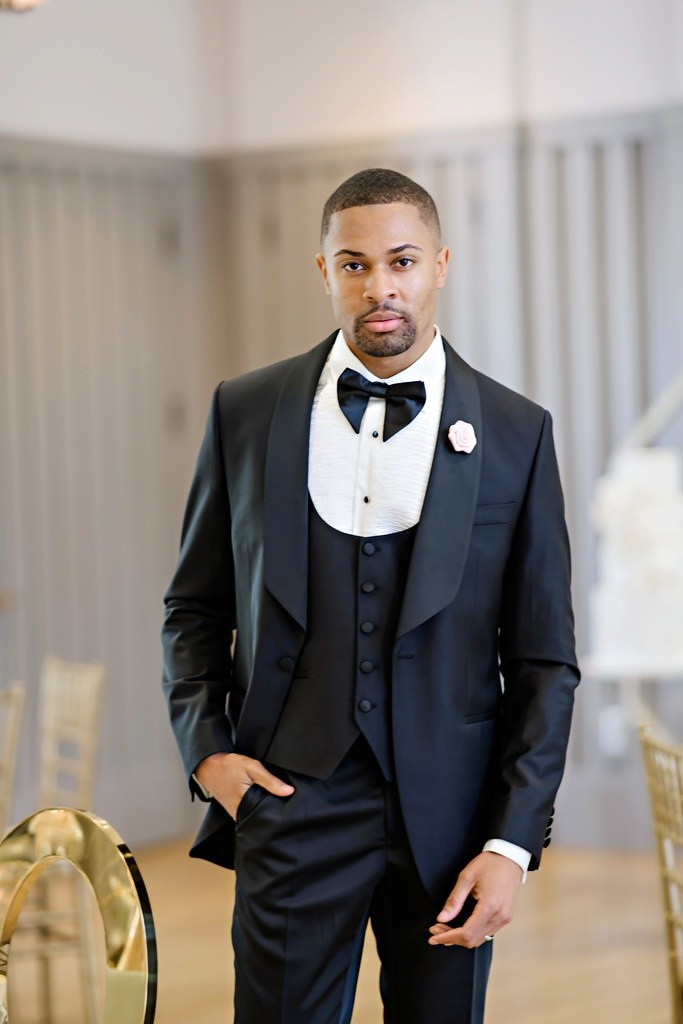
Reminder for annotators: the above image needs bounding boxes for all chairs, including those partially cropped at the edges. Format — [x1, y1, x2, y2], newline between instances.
[638, 717, 682, 1024]
[2, 806, 158, 1024]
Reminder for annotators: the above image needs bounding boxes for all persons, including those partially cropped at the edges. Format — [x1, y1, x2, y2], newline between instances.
[161, 168, 582, 1024]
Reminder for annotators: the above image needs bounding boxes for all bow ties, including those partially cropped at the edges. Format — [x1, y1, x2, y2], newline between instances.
[336, 369, 426, 442]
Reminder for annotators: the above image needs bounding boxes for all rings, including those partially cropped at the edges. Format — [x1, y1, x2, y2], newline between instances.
[485, 935, 494, 941]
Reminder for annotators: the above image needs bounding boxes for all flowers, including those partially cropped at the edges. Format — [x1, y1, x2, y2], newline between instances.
[446, 419, 478, 455]
